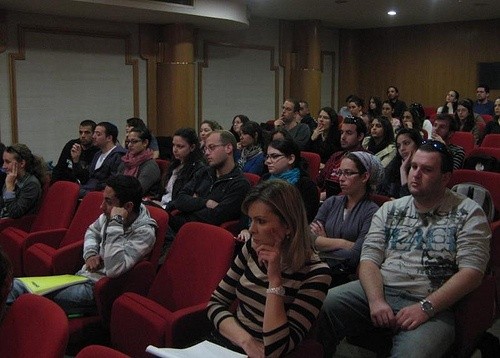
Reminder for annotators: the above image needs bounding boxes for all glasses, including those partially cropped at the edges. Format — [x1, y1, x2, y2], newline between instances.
[421, 139, 450, 163]
[345, 115, 359, 125]
[201, 144, 226, 153]
[4, 146, 24, 160]
[121, 139, 143, 146]
[265, 154, 286, 160]
[336, 169, 363, 178]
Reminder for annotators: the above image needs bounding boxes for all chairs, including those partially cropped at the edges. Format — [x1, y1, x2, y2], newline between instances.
[0, 108, 500, 358]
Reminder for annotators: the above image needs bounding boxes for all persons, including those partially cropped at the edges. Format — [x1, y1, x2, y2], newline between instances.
[0, 82, 500, 358]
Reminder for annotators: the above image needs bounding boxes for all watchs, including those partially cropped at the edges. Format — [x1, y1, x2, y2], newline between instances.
[110, 214, 126, 224]
[266, 286, 286, 296]
[419, 299, 435, 319]
